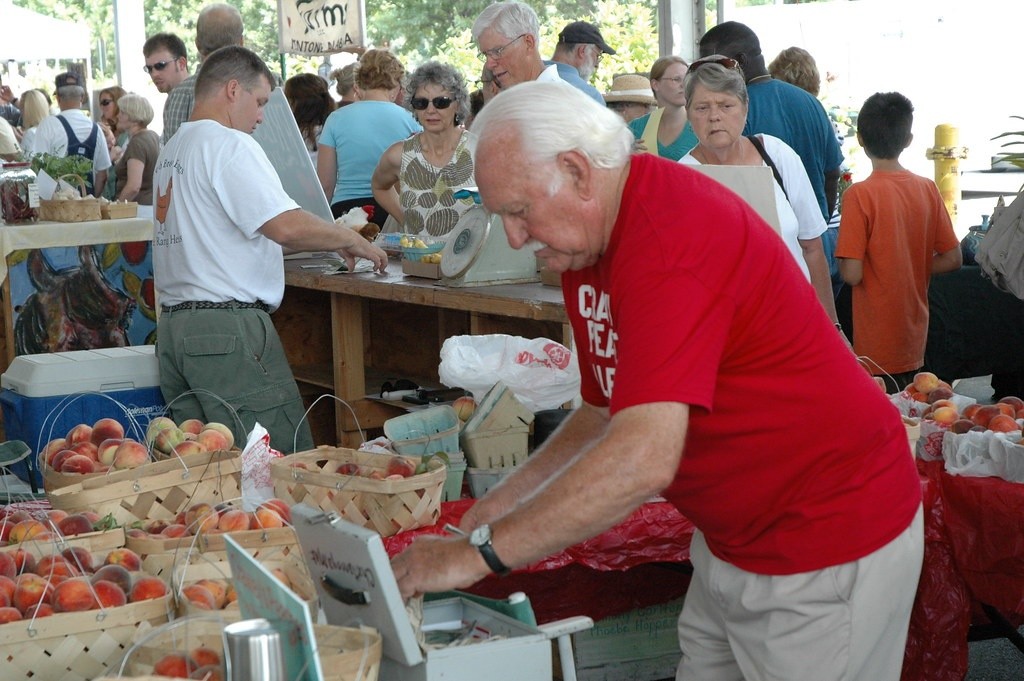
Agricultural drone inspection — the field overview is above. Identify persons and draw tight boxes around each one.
[389,77,924,681]
[32,72,112,199]
[676,54,854,356]
[315,47,424,239]
[834,92,962,397]
[371,61,484,239]
[152,47,388,456]
[0,0,844,282]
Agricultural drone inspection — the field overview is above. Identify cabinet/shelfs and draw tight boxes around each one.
[267,250,579,451]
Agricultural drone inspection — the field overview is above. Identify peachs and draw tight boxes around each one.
[284,454,416,479]
[0,498,291,681]
[40,418,149,474]
[871,371,1024,447]
[453,396,478,421]
[146,416,234,458]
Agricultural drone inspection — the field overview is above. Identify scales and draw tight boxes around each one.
[434,187,544,287]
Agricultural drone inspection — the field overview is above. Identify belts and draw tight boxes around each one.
[161,299,269,312]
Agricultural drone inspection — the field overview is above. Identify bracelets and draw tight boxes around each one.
[11,98,18,104]
[835,322,842,332]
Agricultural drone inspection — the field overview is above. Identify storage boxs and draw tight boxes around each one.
[0,345,175,493]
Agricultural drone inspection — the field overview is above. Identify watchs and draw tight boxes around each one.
[468,524,511,574]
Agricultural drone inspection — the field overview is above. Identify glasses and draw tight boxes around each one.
[660,76,682,84]
[689,58,738,71]
[591,47,604,62]
[143,57,178,74]
[476,34,525,64]
[100,99,114,106]
[410,97,456,110]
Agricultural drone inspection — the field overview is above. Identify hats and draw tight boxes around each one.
[558,20,616,54]
[53,72,83,95]
[604,75,654,104]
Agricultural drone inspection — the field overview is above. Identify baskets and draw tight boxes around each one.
[856,354,921,465]
[1,380,535,681]
[38,174,103,222]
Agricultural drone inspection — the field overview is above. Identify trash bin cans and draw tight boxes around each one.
[0,345,171,488]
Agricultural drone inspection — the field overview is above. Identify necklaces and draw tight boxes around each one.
[128,127,144,138]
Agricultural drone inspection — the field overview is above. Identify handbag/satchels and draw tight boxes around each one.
[440,332,579,413]
[974,181,1024,299]
